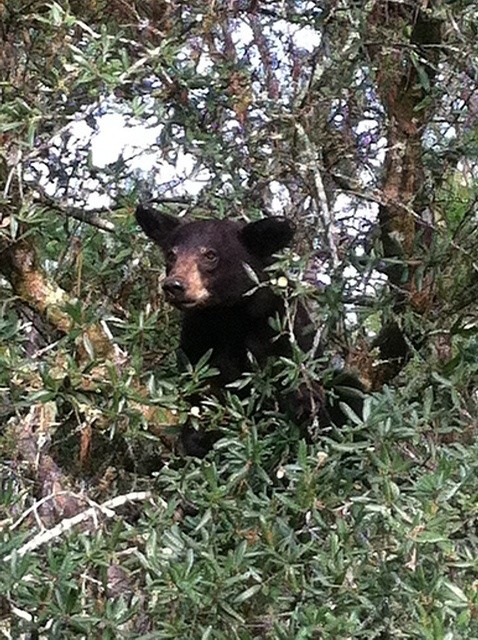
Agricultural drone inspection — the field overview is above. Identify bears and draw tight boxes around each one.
[134,203,372,468]
